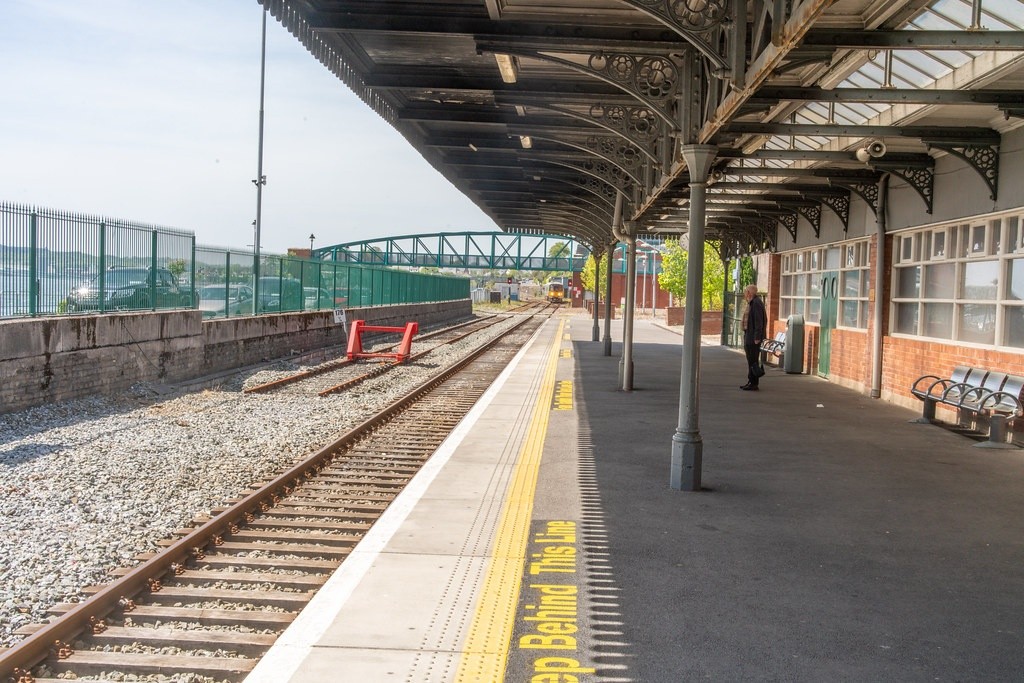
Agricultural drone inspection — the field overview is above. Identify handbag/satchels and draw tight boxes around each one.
[751,364,766,378]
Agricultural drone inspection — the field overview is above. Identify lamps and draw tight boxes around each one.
[495,54,519,83]
[519,136,532,149]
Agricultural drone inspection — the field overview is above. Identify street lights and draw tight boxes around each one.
[309,233,315,258]
[252,174,267,283]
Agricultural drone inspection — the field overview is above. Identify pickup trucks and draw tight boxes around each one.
[65,265,199,310]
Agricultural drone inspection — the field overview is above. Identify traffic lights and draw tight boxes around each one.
[568,279,572,286]
[507,277,512,283]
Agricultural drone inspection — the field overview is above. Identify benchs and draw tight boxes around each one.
[910,366,1024,450]
[761,332,788,368]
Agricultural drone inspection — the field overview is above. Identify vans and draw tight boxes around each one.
[248,277,304,311]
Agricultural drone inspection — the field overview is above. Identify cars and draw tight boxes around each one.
[303,288,334,309]
[329,286,375,304]
[200,284,262,316]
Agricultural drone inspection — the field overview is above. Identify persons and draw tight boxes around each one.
[739,285,767,390]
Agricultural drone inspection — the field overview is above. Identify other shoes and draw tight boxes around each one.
[743,382,759,391]
[740,379,752,389]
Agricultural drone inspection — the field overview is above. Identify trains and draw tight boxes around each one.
[520,282,564,303]
